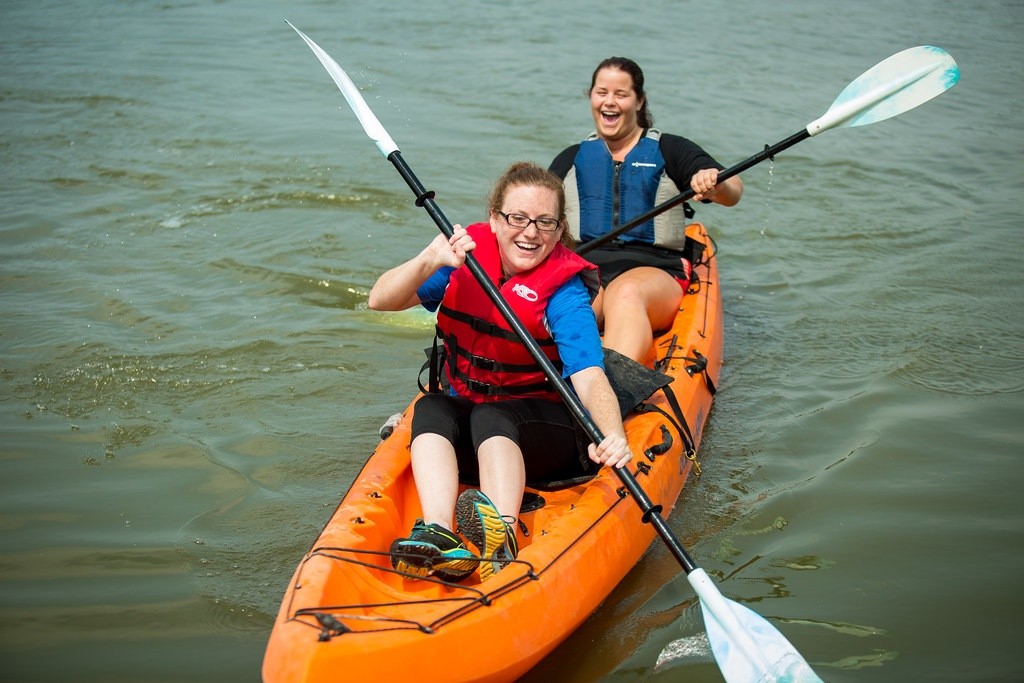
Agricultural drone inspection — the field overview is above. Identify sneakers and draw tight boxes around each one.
[390,518,480,583]
[455,489,519,585]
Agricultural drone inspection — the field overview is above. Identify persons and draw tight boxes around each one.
[546,55,744,372]
[368,160,634,581]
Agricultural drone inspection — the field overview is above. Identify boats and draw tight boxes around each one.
[260,222,723,682]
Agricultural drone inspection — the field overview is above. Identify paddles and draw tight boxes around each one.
[574,43,963,259]
[282,15,823,683]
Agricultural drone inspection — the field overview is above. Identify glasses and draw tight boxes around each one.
[499,210,565,232]
